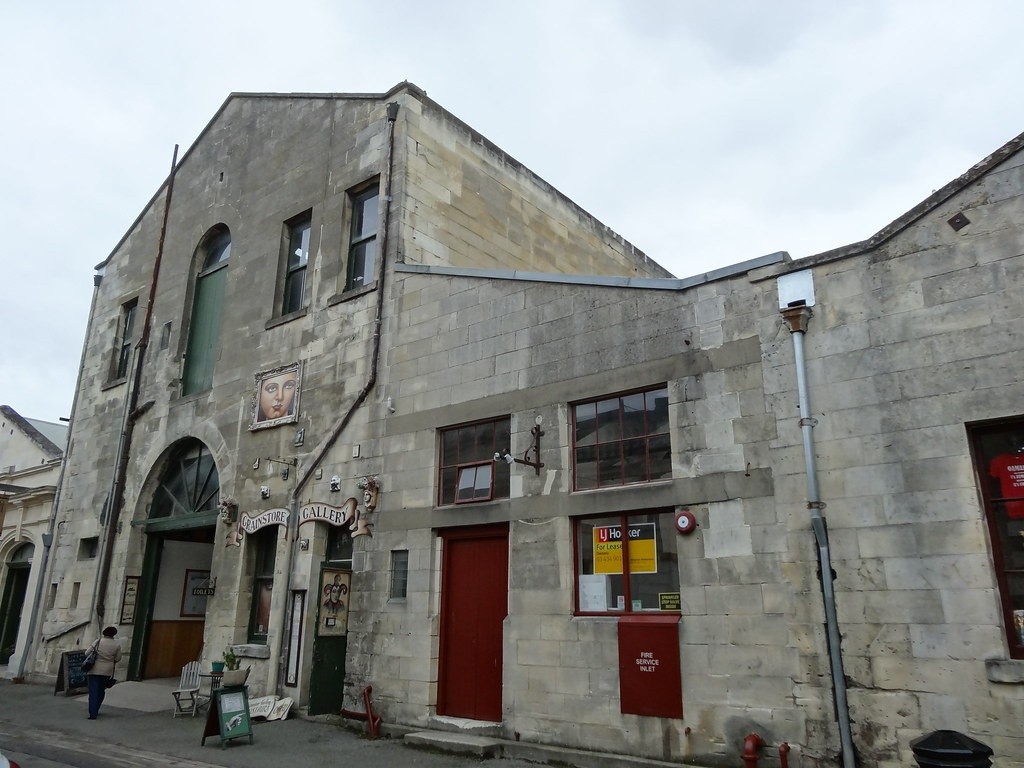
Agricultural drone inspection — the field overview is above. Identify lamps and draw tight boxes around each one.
[494,425,545,476]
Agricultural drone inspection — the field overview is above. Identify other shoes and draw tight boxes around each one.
[88,715,97,720]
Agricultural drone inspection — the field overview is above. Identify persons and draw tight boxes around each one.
[85,626,121,720]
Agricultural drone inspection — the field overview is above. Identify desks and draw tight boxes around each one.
[196,672,224,714]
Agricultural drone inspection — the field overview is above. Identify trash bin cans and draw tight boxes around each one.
[909,729,994,768]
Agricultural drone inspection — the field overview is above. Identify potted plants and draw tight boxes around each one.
[212,660,225,672]
[220,647,246,686]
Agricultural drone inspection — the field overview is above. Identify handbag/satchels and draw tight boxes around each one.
[81,651,98,672]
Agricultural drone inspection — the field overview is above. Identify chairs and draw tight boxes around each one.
[172,661,203,718]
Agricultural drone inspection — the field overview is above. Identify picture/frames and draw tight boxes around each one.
[284,589,308,688]
[318,571,350,636]
[247,362,304,431]
[179,569,211,617]
[120,576,141,624]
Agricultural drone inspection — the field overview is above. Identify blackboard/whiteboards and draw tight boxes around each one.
[62,648,90,695]
